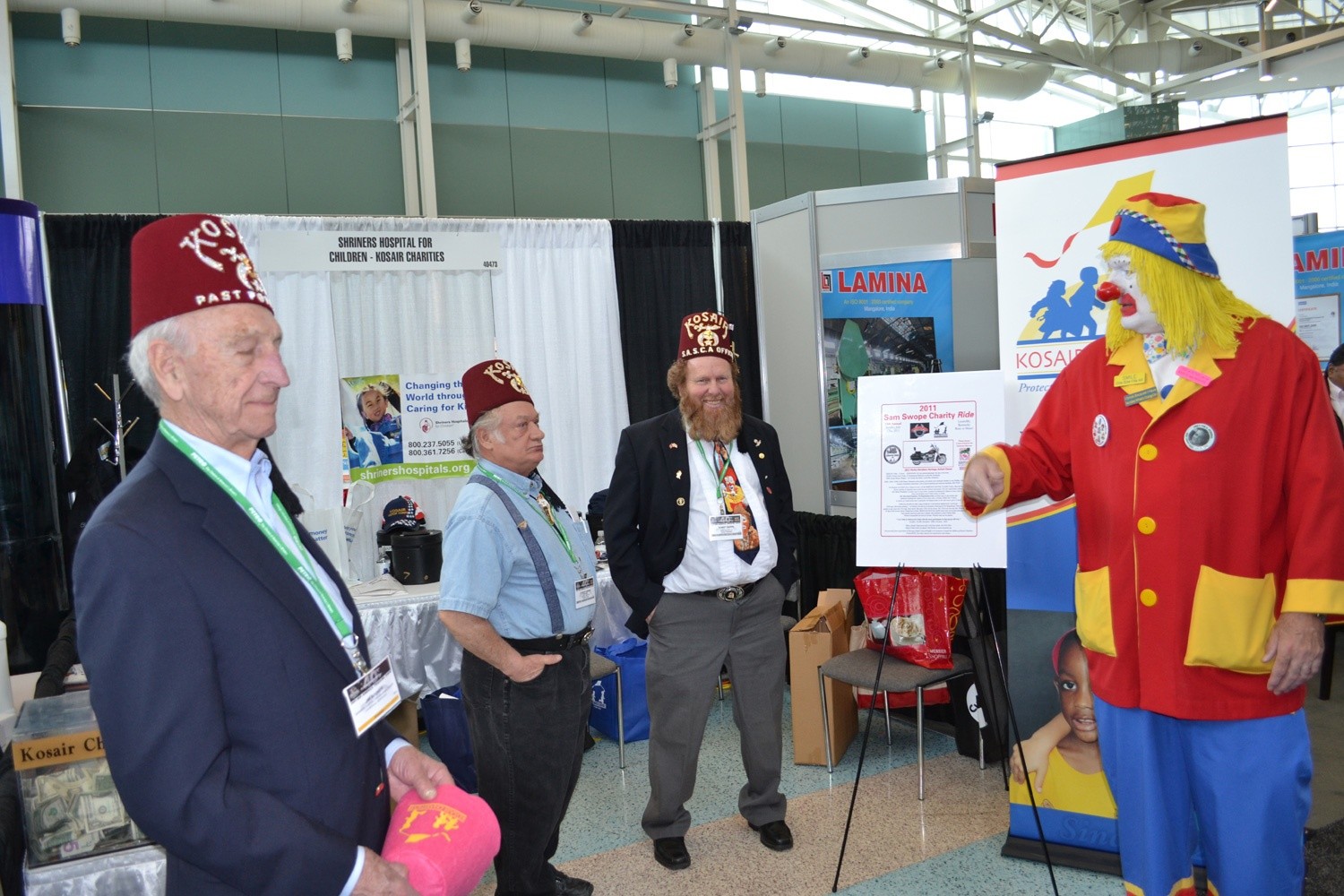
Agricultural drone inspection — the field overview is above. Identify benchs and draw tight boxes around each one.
[775,512,878,627]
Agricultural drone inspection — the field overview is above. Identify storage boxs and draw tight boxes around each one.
[10,689,163,866]
[787,585,861,769]
[19,844,169,896]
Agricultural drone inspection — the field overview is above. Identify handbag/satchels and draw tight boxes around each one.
[854,567,967,664]
[585,487,611,540]
[419,680,481,797]
[392,527,444,583]
[589,639,653,745]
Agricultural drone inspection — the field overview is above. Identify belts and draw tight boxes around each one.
[695,577,765,603]
[502,621,596,658]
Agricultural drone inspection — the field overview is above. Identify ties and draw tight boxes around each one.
[709,437,762,566]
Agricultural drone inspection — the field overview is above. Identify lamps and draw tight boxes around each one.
[921,58,944,75]
[662,59,678,89]
[344,0,358,12]
[462,0,483,24]
[573,11,592,32]
[335,29,354,63]
[60,9,81,48]
[764,36,787,55]
[849,46,870,65]
[674,23,694,45]
[455,39,471,71]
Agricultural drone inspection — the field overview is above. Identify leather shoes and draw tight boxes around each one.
[653,837,691,870]
[547,867,594,895]
[748,817,793,852]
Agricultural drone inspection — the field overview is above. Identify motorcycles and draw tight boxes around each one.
[909,444,947,466]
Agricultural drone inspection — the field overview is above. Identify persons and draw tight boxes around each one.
[604,337,802,870]
[73,274,456,895]
[1322,343,1344,444]
[1008,622,1118,819]
[963,195,1344,896]
[341,382,403,469]
[436,391,598,896]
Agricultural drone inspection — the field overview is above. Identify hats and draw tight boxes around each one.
[462,361,535,426]
[383,784,503,896]
[1112,193,1221,279]
[123,213,276,337]
[676,309,735,364]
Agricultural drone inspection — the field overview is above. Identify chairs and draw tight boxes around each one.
[586,650,626,772]
[817,649,986,802]
